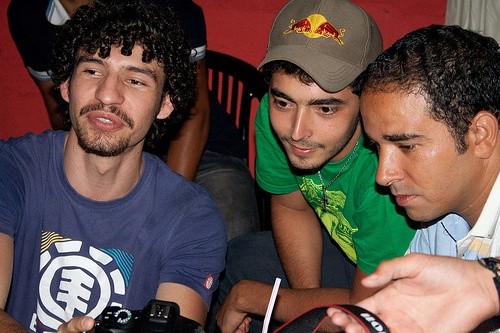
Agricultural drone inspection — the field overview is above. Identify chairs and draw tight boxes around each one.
[201,49,271,176]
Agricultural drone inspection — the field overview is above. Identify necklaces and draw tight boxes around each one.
[317,132,368,215]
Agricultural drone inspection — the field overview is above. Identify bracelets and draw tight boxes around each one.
[480,257,500,304]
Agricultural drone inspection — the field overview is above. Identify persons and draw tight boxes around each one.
[351,24,500,333]
[214,1,421,333]
[6,0,210,181]
[327,253,500,332]
[1,1,226,333]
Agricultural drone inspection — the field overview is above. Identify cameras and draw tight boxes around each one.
[94,299,202,333]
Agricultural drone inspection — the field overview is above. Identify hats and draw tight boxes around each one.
[257,0,383,94]
